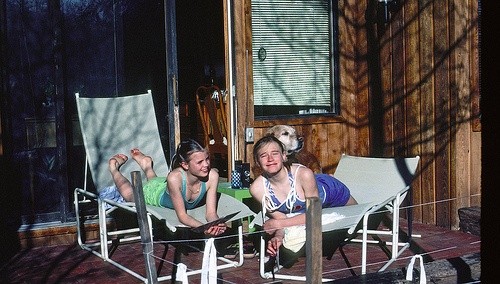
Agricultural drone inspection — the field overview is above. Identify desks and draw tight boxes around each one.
[199,99,230,148]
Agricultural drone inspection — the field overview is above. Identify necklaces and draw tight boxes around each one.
[187,182,202,195]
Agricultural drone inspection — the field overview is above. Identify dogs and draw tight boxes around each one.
[251,125,320,179]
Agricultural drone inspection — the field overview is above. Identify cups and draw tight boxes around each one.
[230,160,251,189]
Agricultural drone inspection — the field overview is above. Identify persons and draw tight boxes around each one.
[108,137,227,236]
[249,135,358,257]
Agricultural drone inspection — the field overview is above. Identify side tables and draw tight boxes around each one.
[216,180,261,250]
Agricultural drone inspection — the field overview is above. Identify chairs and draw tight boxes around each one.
[248,153,421,284]
[72,87,255,283]
[195,84,229,171]
